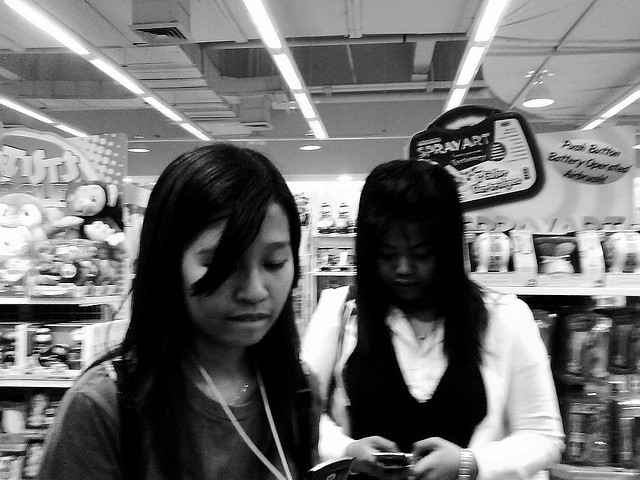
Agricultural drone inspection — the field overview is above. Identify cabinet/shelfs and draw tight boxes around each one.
[1,293,131,390]
[305,233,358,308]
[470,271,640,479]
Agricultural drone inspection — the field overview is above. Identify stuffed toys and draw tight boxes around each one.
[474,223,519,278]
[66,180,126,247]
[27,327,75,363]
[0,193,85,285]
[22,397,54,431]
[332,201,350,233]
[316,202,335,234]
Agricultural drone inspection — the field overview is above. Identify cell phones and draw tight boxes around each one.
[306,456,357,480]
[370,452,413,466]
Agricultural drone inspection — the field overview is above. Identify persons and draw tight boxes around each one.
[299,158,567,480]
[34,142,320,480]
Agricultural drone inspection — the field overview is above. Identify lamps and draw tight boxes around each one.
[0,96,89,138]
[242,1,329,141]
[523,74,554,109]
[127,137,151,154]
[1,1,216,142]
[299,133,321,151]
[577,82,640,129]
[442,0,512,111]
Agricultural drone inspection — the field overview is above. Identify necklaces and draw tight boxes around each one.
[413,322,435,342]
[226,375,253,405]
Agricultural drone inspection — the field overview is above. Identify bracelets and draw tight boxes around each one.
[458,448,474,480]
